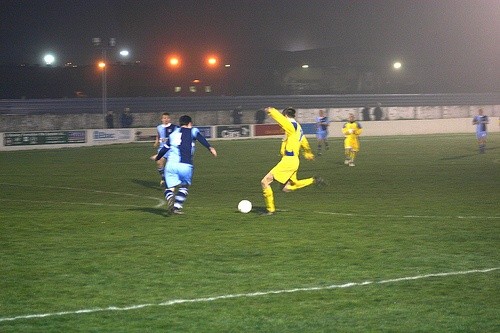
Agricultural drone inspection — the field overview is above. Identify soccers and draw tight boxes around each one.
[237,199,253,214]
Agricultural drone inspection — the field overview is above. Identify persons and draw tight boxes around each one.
[153,113,180,192]
[362,103,372,120]
[155,115,217,215]
[255,107,266,123]
[119,107,133,128]
[105,109,115,128]
[260,106,330,216]
[473,109,489,153]
[373,102,383,120]
[316,110,329,157]
[342,113,363,167]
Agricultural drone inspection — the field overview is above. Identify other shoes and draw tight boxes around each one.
[261,210,276,216]
[312,176,322,185]
[344,158,352,164]
[349,162,356,167]
[160,180,166,187]
[172,207,186,215]
[167,200,176,215]
